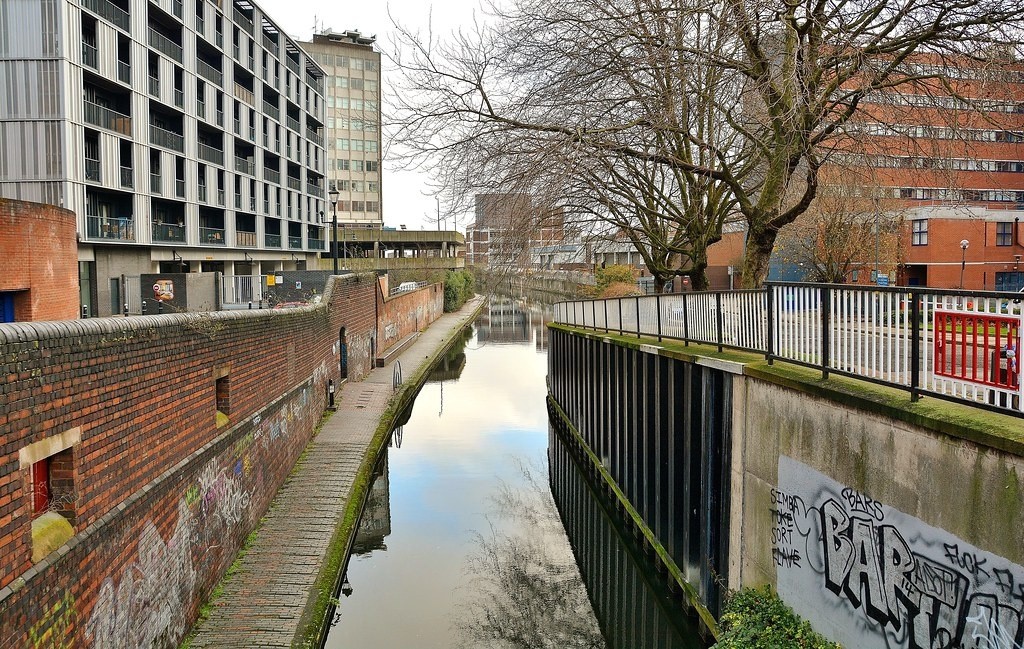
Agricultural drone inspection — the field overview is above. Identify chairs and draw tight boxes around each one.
[99,225,118,239]
[169,230,174,241]
[208,233,223,244]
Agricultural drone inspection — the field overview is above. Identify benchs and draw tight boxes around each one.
[377,331,419,367]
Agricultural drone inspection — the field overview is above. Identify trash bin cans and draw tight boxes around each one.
[989,345,1019,409]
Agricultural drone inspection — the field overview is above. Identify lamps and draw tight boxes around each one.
[329,385,335,394]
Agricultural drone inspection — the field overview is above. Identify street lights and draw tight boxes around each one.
[328,184,340,275]
[957,239,970,307]
[1013,254,1023,292]
